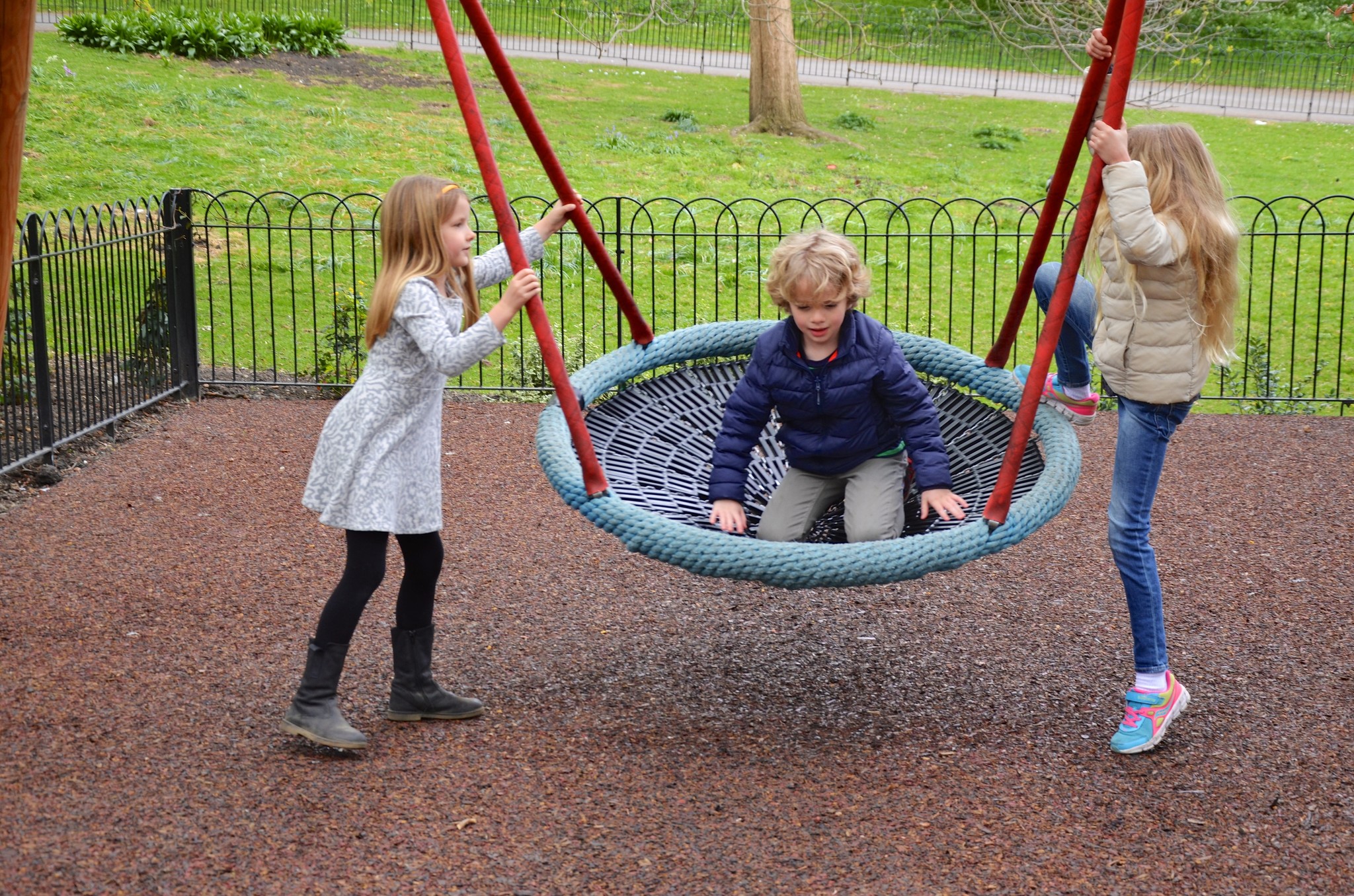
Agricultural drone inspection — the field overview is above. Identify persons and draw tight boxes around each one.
[1014,27,1243,754]
[282,176,583,749]
[707,230,969,544]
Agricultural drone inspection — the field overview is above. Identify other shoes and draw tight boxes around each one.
[905,459,913,501]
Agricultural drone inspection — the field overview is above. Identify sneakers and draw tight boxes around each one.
[1013,365,1098,425]
[1110,670,1191,754]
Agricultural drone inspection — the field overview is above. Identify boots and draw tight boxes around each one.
[387,622,486,721]
[278,636,369,748]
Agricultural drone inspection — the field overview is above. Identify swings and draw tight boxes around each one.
[421,1,1149,592]
